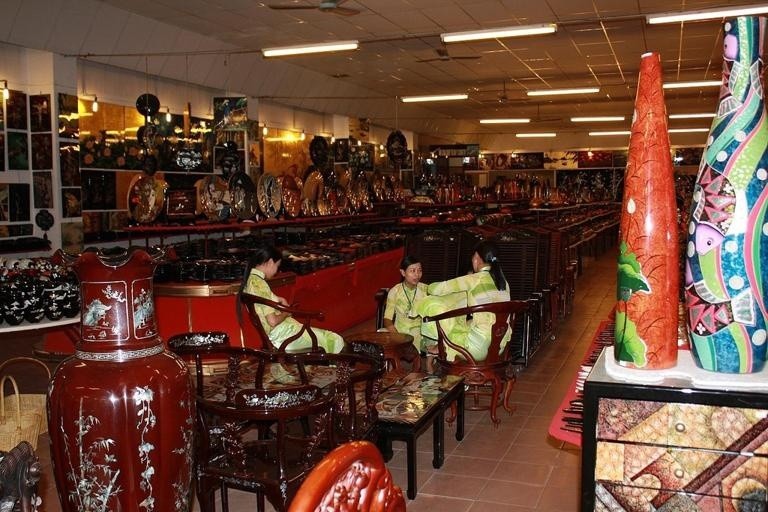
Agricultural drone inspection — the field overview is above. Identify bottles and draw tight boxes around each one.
[529,186,563,208]
[496,185,501,200]
[521,184,525,198]
[353,145,373,169]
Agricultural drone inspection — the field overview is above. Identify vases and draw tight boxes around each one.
[44,244,199,511]
[684,15,767,372]
[615,52,680,370]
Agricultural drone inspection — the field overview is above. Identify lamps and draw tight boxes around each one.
[261,4,766,59]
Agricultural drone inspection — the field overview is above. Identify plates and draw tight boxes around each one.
[129,175,164,224]
[200,170,404,222]
[309,136,329,167]
[386,130,408,165]
[176,148,203,170]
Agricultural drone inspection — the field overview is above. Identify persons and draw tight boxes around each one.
[236,245,345,354]
[375,256,429,356]
[408,240,513,362]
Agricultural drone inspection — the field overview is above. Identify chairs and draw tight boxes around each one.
[181,331,466,512]
[424,300,527,427]
[239,292,330,365]
